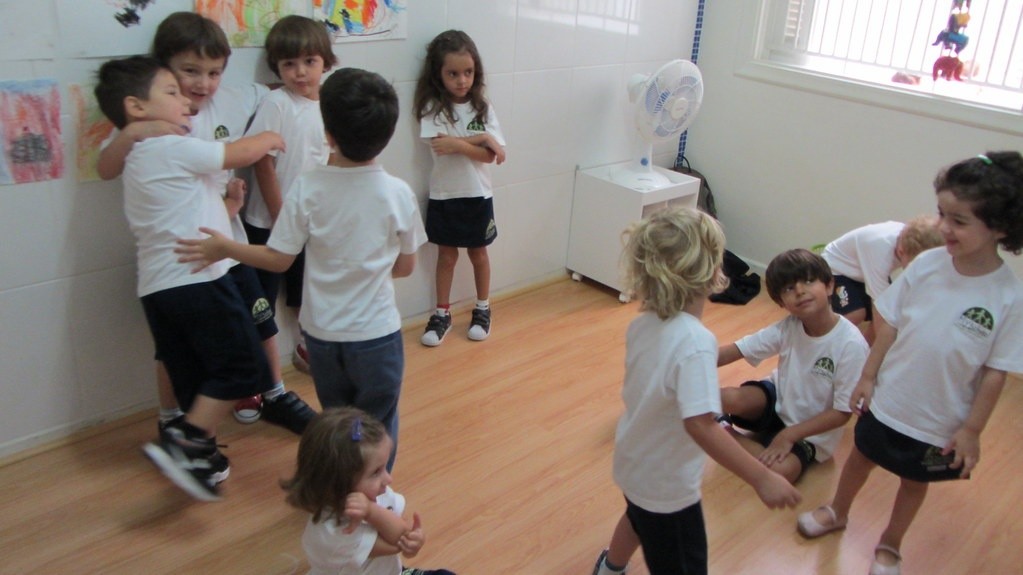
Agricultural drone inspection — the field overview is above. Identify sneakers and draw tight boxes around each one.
[261,388,322,434]
[420,311,452,347]
[466,305,492,341]
[142,422,220,504]
[158,413,232,481]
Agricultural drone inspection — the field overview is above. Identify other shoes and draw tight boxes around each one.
[291,344,310,375]
[797,504,847,538]
[234,392,263,423]
[869,544,903,575]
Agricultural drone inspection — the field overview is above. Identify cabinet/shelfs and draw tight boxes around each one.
[565,159,702,301]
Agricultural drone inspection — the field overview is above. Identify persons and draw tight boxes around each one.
[796,150,1022,575]
[412,29,506,347]
[171,66,428,510]
[92,10,428,501]
[593,206,802,575]
[819,214,946,326]
[717,249,871,486]
[276,406,456,575]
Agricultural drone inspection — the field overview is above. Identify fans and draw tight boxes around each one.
[625,58,704,189]
